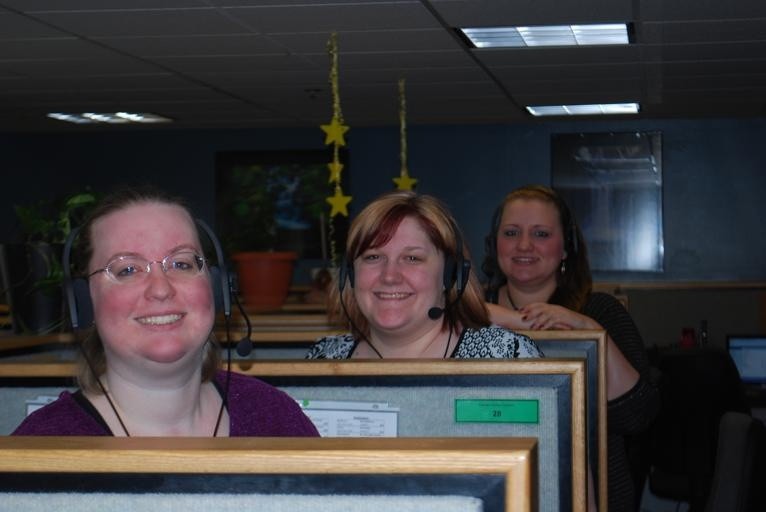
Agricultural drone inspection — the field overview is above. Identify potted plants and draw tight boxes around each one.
[5,196,93,336]
[214,164,313,313]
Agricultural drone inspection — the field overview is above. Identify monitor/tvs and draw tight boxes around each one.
[726,333,766,384]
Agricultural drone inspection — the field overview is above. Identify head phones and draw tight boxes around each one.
[484,201,582,262]
[62,213,245,330]
[338,213,471,294]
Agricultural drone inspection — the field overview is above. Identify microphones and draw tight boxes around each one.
[428,286,465,320]
[232,295,253,357]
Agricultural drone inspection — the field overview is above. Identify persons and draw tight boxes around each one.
[7,181,323,437]
[304,190,545,360]
[482,183,663,511]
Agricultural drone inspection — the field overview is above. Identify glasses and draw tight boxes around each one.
[85,252,209,284]
